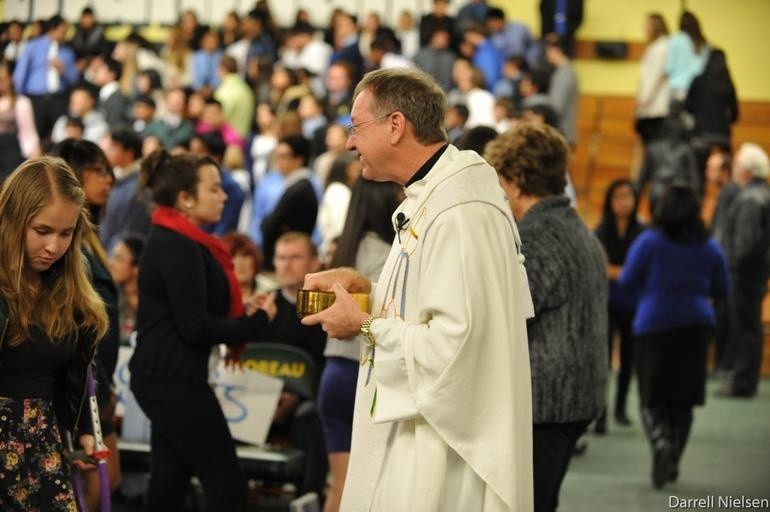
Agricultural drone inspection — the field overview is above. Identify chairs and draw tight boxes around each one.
[102,344,152,461]
[207,337,316,500]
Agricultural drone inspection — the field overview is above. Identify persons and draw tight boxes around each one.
[130,148,278,510]
[482,121,610,512]
[318,175,405,510]
[0,156,110,510]
[1,3,769,488]
[299,66,539,510]
